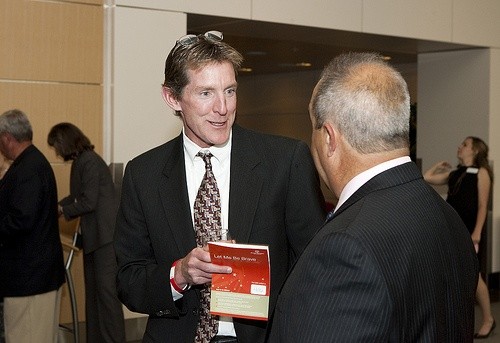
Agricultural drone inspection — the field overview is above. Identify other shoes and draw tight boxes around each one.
[477,323,496,338]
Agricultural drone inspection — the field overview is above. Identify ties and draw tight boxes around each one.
[194,152,223,343]
[324,209,334,221]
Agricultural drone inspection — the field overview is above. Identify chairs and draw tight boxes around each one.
[59,214,80,343]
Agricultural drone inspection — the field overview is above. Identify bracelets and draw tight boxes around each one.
[171,258,191,293]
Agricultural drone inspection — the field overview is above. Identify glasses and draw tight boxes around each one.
[171,30,224,57]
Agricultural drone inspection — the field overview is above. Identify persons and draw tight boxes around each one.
[114,34,328,343]
[46,122,128,343]
[423,136,497,338]
[265,48,475,343]
[0,111,65,343]
